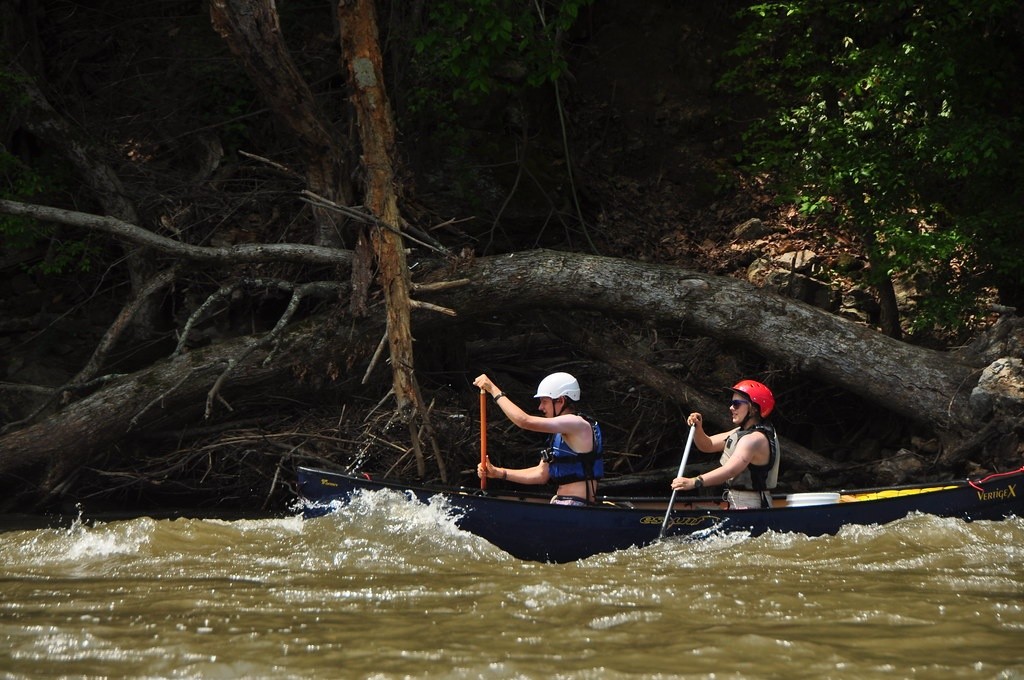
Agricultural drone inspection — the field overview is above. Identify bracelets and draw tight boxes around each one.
[493,392,507,404]
[501,467,507,480]
[697,476,705,483]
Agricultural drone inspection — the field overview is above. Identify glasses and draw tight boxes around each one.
[730,399,749,408]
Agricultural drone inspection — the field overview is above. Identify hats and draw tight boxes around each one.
[732,380,774,417]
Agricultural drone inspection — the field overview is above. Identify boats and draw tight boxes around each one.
[296,464,1024,565]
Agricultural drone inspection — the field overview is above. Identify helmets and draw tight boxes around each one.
[534,372,580,402]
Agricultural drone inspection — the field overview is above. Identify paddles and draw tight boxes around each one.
[655,424,696,541]
[480,390,488,490]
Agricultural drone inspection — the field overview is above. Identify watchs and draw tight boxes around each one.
[693,477,704,490]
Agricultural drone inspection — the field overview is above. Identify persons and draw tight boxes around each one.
[671,380,780,509]
[473,372,603,507]
